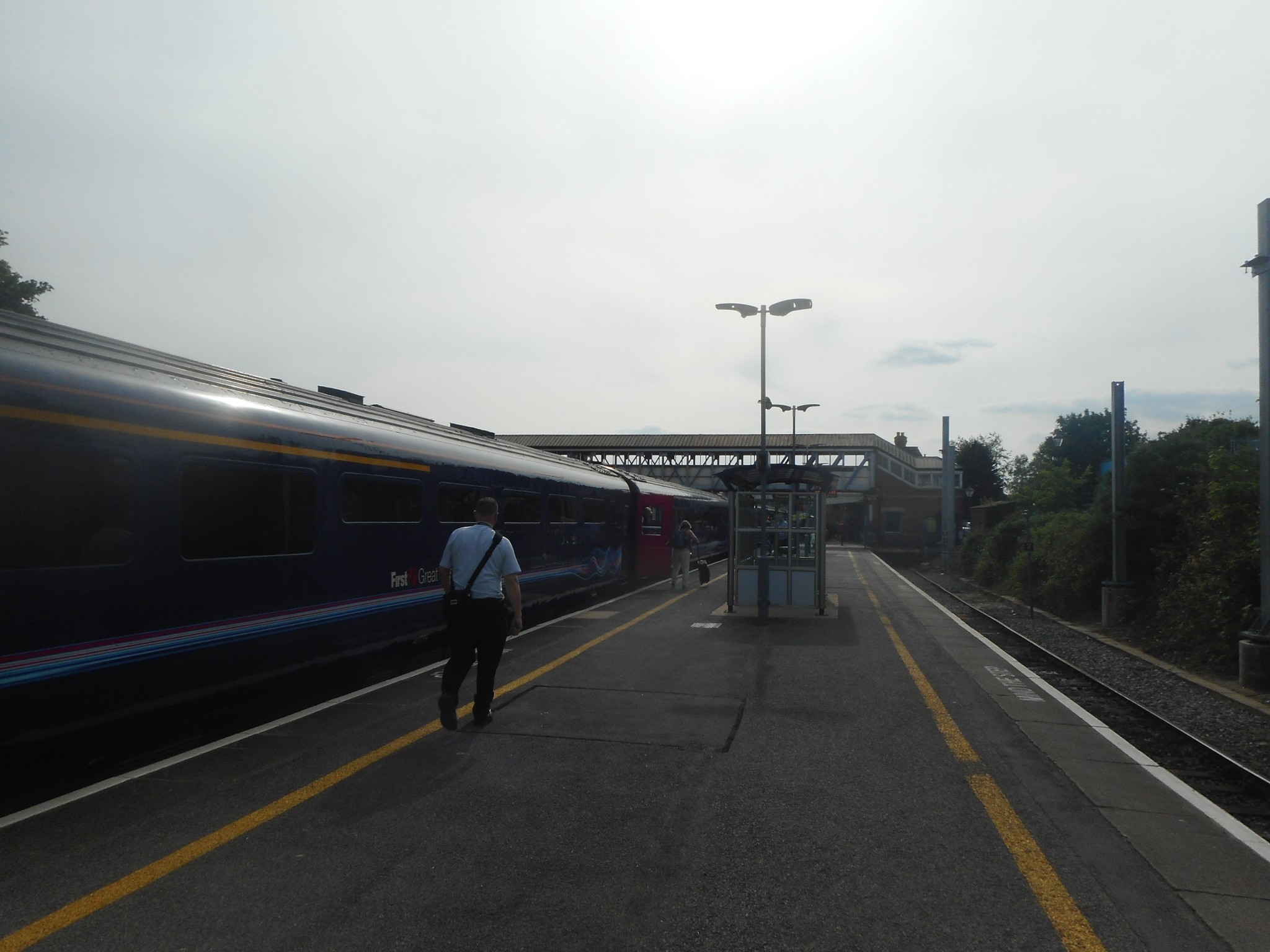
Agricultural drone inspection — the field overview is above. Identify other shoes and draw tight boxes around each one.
[683,584,689,588]
[671,582,675,587]
[438,693,459,732]
[473,711,494,726]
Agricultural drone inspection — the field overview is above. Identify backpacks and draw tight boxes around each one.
[672,528,689,549]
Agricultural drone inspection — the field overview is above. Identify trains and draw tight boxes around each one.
[1,305,735,775]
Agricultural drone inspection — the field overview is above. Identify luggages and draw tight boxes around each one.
[696,543,710,585]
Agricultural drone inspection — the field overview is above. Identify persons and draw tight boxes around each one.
[670,520,700,590]
[436,493,524,729]
[771,511,790,548]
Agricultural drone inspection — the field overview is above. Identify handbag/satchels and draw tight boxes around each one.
[444,590,473,630]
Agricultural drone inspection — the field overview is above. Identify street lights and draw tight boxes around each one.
[715,297,813,628]
[768,403,820,559]
